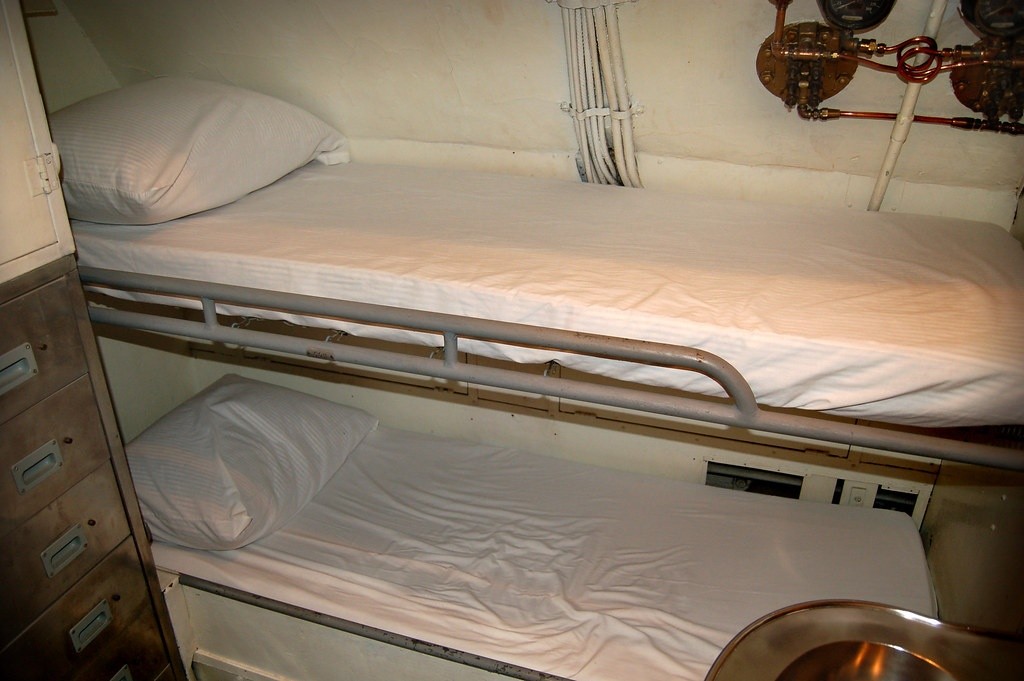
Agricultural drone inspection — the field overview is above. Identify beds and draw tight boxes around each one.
[70,143,1024,681]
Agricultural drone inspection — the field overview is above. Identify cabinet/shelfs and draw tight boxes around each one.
[0,251,188,681]
[0,0,77,283]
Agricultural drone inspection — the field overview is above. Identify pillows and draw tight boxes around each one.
[124,373,381,550]
[46,78,350,226]
